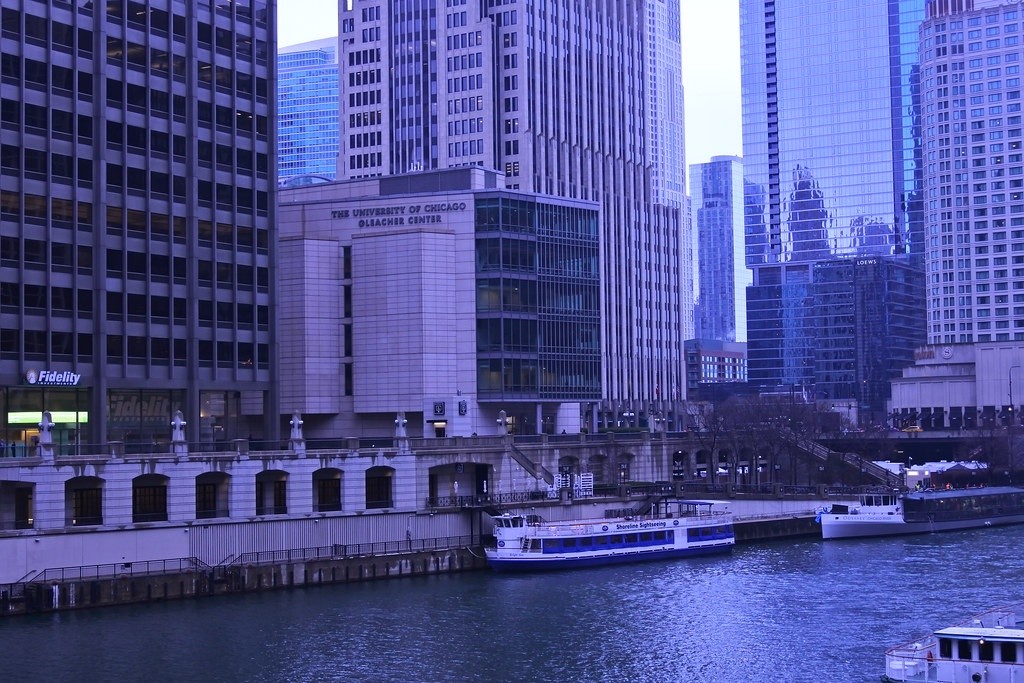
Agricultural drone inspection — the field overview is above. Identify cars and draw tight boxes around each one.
[902,426,923,432]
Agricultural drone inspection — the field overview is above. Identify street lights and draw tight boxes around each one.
[1008,365,1021,404]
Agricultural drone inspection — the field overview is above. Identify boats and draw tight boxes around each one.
[482,502,737,571]
[818,486,1024,540]
[879,604,1024,682]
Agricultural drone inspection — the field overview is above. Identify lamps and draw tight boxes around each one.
[430,514,434,519]
[315,519,319,524]
[531,507,535,511]
[184,528,188,533]
[725,505,728,510]
[593,503,597,506]
[35,536,40,543]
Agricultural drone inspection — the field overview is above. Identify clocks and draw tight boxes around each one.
[26,368,38,384]
